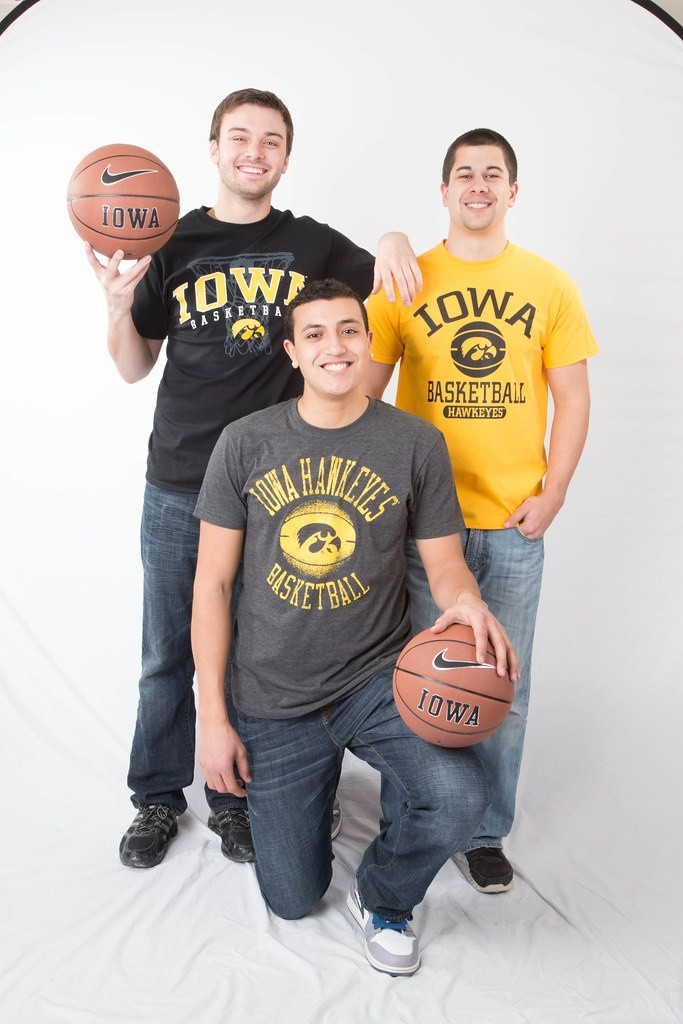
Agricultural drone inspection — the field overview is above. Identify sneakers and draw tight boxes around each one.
[119,803,178,868]
[450,847,514,894]
[329,795,343,840]
[347,866,421,977]
[207,808,256,863]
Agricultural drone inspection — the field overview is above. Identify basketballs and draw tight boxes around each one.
[392,624,515,749]
[67,144,181,261]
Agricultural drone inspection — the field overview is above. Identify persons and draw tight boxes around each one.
[363,128,590,895]
[84,88,424,869]
[190,278,522,974]
